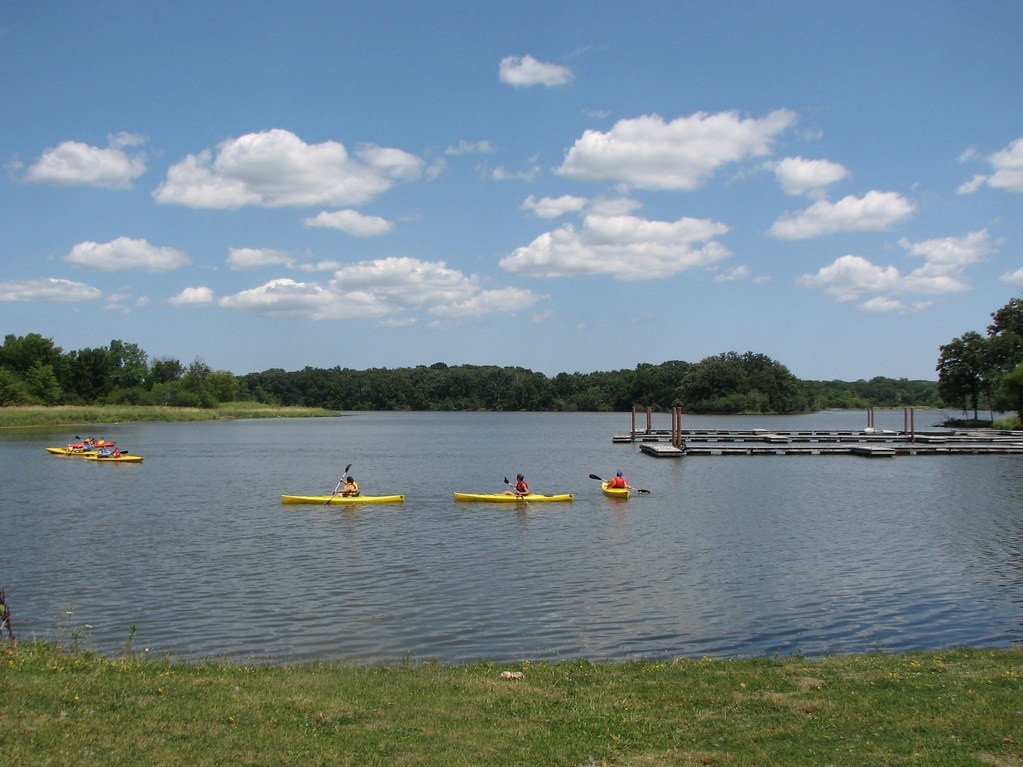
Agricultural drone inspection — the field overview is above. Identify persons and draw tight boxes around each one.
[112,448,122,457]
[607,471,631,490]
[86,436,97,445]
[82,440,93,451]
[97,437,105,445]
[503,473,529,497]
[334,476,361,498]
[98,446,111,456]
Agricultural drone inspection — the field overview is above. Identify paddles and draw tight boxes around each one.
[323,464,353,503]
[590,474,652,494]
[503,477,525,500]
[75,436,84,441]
[120,450,129,454]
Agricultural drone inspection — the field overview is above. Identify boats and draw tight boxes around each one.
[453,492,574,503]
[281,494,405,504]
[68,441,116,448]
[601,481,630,499]
[46,447,98,455]
[83,455,144,463]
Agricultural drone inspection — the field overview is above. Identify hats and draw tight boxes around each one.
[617,471,623,477]
